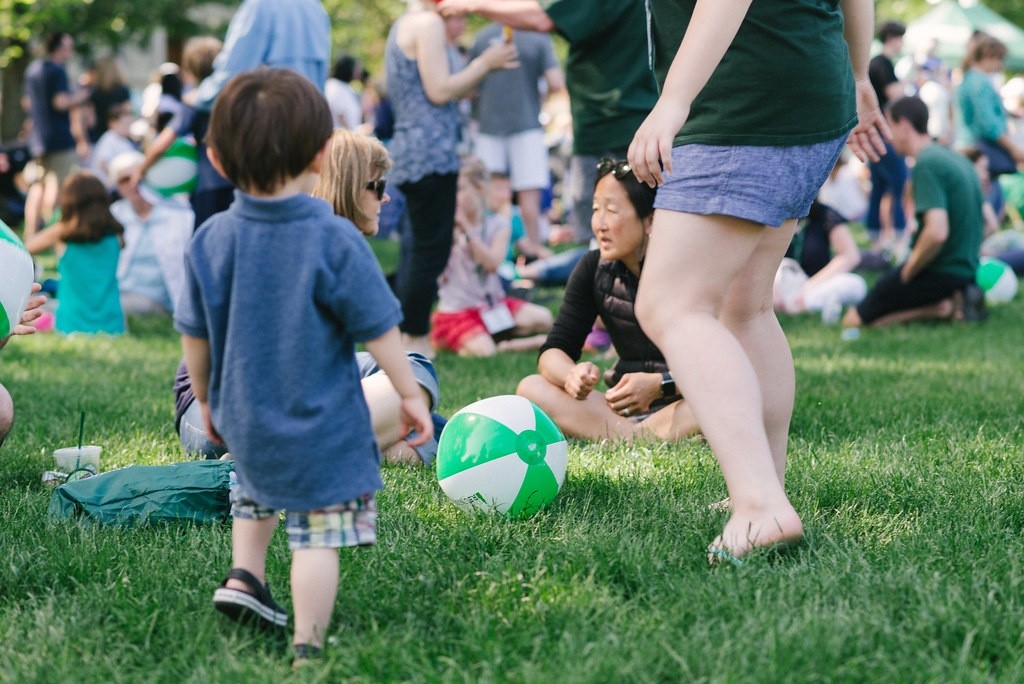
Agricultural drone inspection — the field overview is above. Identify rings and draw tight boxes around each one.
[624,408,630,417]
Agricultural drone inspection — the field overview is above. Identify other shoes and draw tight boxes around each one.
[953,284,986,326]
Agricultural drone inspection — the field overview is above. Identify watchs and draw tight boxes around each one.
[660,370,677,401]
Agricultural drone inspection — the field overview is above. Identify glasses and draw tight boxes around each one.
[364,180,386,200]
[595,157,631,180]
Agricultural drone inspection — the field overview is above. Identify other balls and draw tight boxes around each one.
[145,135,200,204]
[972,259,1020,310]
[435,394,573,524]
[0,216,35,341]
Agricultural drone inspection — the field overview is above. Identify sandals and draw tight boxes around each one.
[290,644,322,670]
[213,571,288,631]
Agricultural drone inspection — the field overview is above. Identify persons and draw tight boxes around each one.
[431,156,557,355]
[626,0,894,564]
[0,0,1023,325]
[174,69,434,670]
[14,159,132,338]
[517,159,702,446]
[174,126,449,465]
[0,283,46,445]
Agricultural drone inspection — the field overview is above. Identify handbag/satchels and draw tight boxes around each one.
[47,458,231,527]
